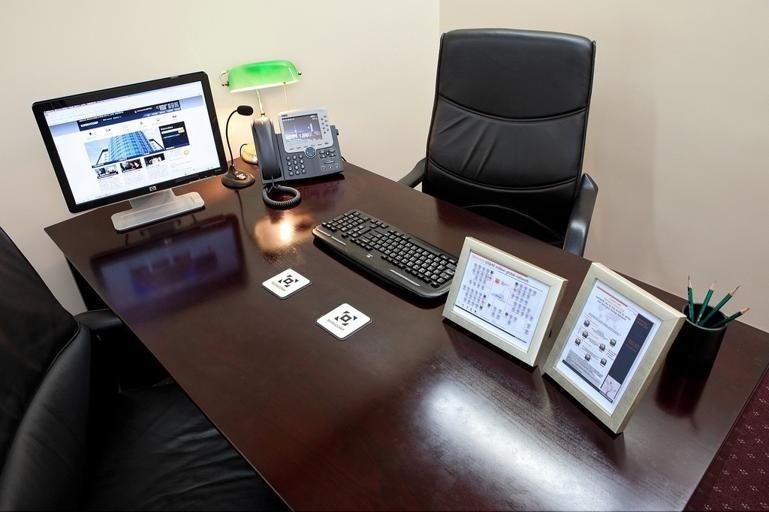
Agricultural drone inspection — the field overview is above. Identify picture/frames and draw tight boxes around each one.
[543,262,688,434]
[442,237,569,367]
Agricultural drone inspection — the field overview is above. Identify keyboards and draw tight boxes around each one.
[313,208,456,305]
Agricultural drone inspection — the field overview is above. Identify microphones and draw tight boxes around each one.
[223,104,255,188]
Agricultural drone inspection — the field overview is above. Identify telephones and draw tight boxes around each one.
[251,107,344,209]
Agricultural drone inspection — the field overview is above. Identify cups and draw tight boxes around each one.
[656,302,728,417]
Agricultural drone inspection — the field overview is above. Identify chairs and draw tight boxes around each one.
[0,226,293,511]
[397,28,599,258]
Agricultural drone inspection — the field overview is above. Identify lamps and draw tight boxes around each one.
[220,60,301,163]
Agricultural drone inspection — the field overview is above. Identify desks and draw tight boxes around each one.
[44,156,768,511]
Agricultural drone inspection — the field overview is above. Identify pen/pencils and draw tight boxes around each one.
[695,280,716,325]
[712,307,750,327]
[700,285,740,325]
[687,276,695,323]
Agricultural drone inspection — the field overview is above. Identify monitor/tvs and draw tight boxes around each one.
[29,70,227,233]
